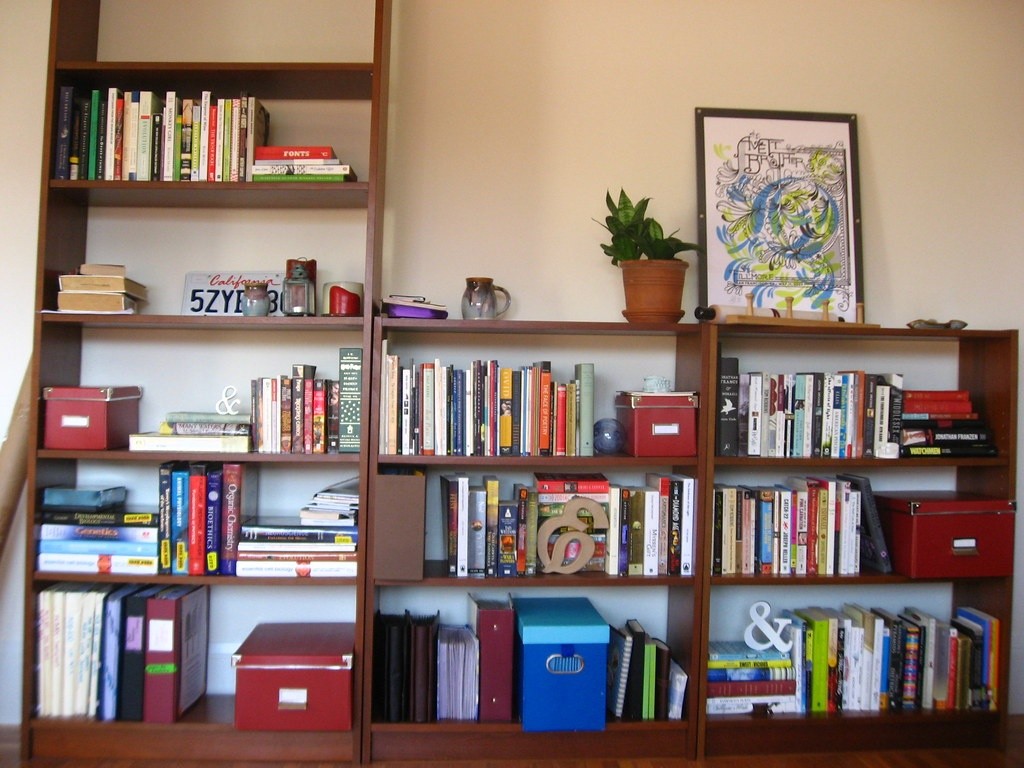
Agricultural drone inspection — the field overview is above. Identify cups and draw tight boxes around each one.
[461,277,512,320]
[321,282,363,317]
[329,285,360,317]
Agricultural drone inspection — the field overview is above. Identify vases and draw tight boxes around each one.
[459,275,510,321]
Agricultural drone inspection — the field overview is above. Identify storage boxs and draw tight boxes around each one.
[43,385,140,450]
[614,393,696,457]
[868,489,1016,578]
[509,597,611,732]
[229,622,354,733]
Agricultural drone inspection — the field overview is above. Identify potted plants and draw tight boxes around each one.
[598,188,693,325]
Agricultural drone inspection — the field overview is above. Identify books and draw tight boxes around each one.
[54,84,359,181]
[125,346,361,455]
[711,475,895,576]
[35,460,360,578]
[377,337,703,579]
[34,583,208,724]
[55,261,150,313]
[714,355,999,457]
[706,601,1001,710]
[372,591,689,724]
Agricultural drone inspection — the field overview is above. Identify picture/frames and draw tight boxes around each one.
[692,104,866,324]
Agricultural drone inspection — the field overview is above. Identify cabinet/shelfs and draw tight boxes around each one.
[1,1,1017,767]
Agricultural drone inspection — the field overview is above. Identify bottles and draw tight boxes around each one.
[240,282,271,317]
